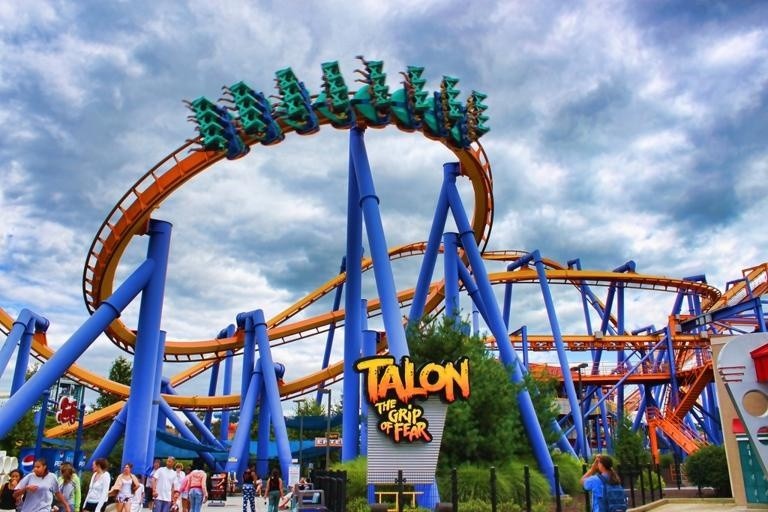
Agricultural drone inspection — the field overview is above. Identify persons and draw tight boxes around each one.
[0,452,209,512]
[581,453,621,512]
[241,464,309,511]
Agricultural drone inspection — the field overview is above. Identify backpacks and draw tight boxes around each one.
[597,474,628,512]
